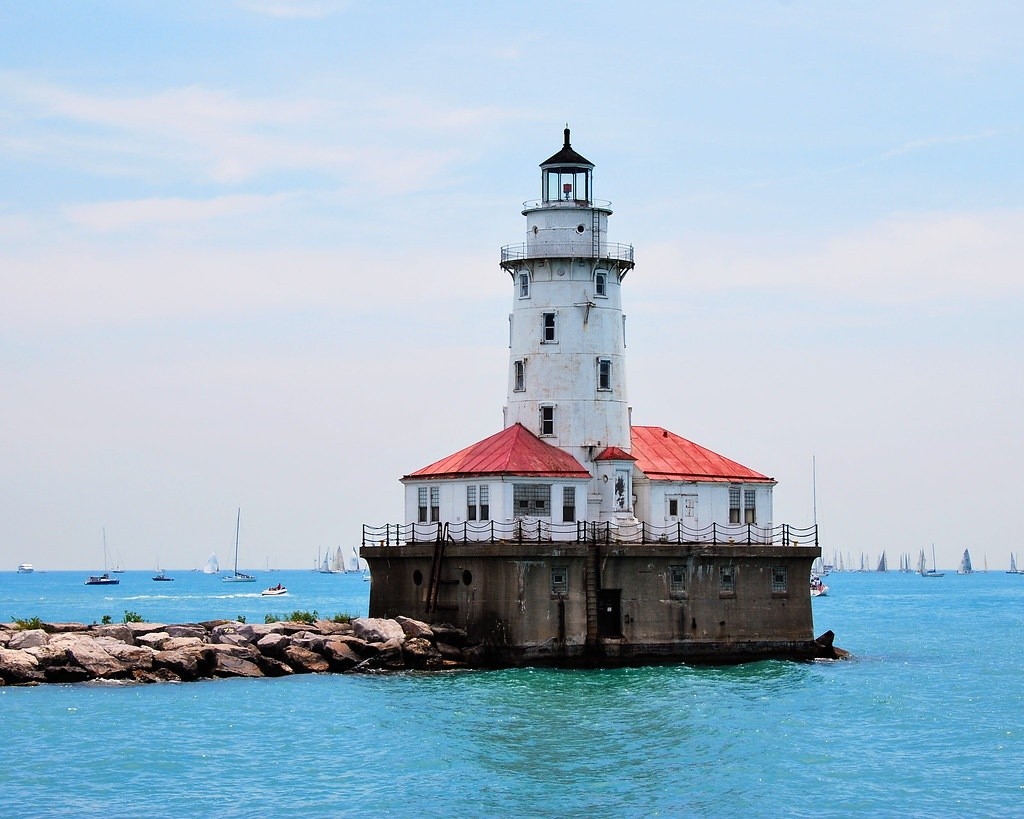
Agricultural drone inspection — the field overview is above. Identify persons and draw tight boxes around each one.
[276,583,282,591]
[810,577,822,590]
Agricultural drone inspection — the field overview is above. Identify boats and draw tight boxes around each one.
[810,573,829,596]
[16,563,34,574]
[110,566,127,574]
[260,587,288,596]
[151,573,175,582]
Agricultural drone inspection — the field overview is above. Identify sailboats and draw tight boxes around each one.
[311,543,372,582]
[203,551,221,574]
[811,546,928,575]
[83,527,120,585]
[221,505,258,582]
[262,555,275,571]
[921,544,946,577]
[1005,552,1023,574]
[809,455,829,577]
[956,547,975,576]
